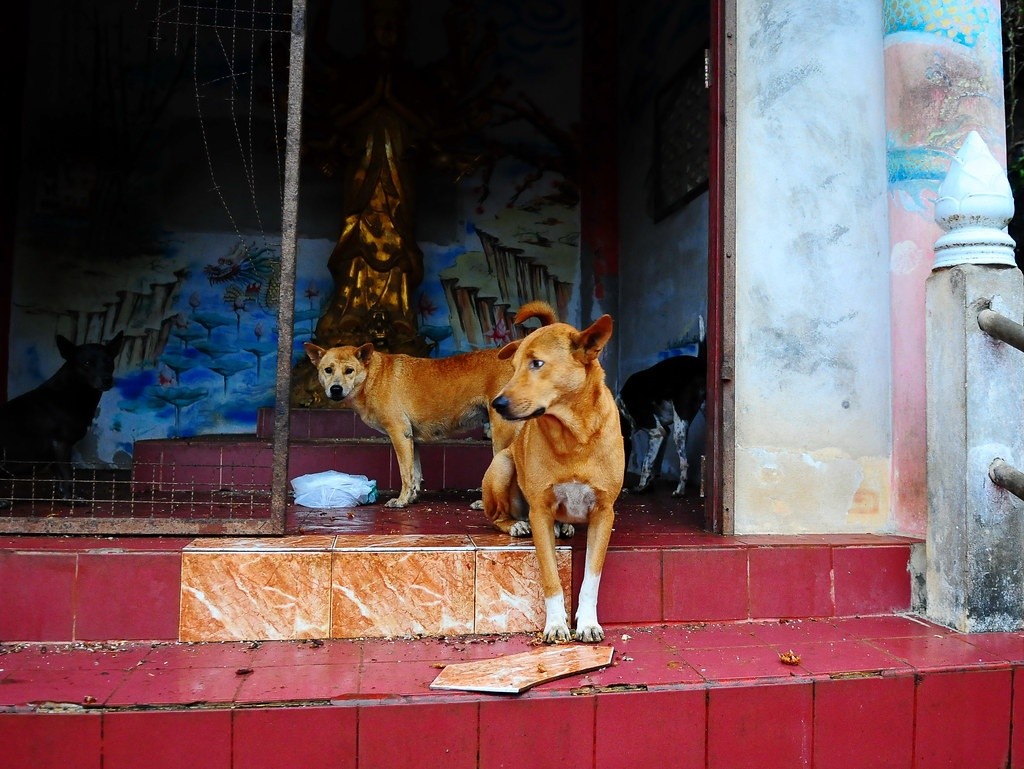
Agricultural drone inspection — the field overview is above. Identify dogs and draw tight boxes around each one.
[0,329,127,511]
[302,343,528,511]
[482,299,626,644]
[614,314,707,497]
